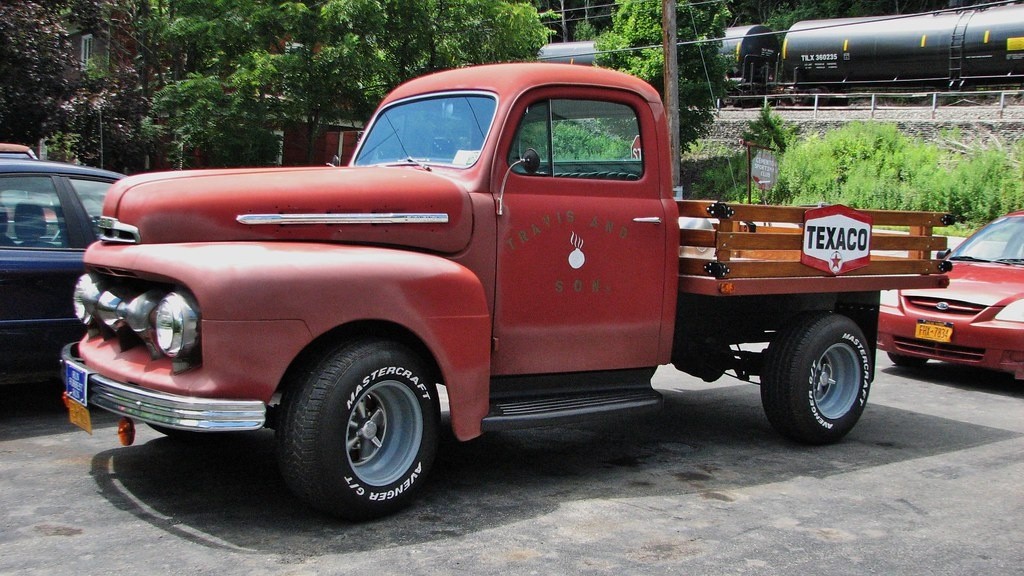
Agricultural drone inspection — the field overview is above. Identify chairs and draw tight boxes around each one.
[0,204,16,246]
[14,203,55,247]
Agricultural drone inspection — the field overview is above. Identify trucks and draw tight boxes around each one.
[57,61,954,521]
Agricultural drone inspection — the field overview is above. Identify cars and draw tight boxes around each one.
[875,209,1024,380]
[0,158,130,363]
[0,143,59,239]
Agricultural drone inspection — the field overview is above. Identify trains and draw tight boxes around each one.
[537,0,1024,107]
